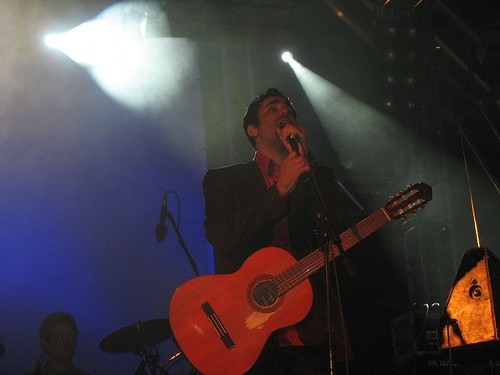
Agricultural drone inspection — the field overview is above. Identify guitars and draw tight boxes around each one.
[169,182,433,375]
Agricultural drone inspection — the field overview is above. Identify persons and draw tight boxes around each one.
[28,312,85,375]
[203,88,377,375]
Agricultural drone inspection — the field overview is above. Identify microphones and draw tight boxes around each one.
[277,122,299,151]
[155,193,168,242]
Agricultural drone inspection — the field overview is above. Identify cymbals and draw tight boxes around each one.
[98,317,172,354]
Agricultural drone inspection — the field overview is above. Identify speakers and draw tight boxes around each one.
[439,246,500,350]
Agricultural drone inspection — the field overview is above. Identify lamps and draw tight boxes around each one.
[378,0,442,124]
